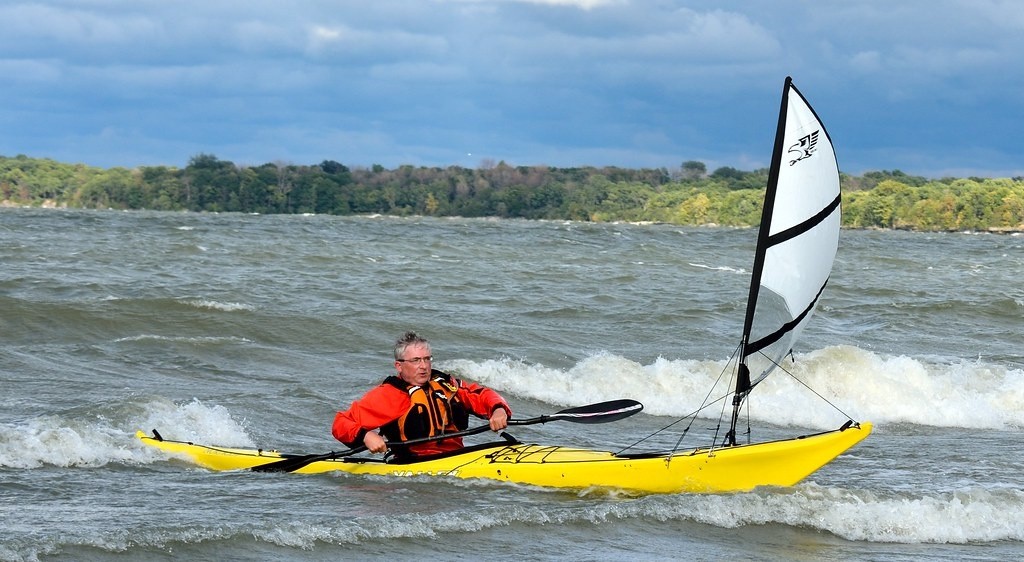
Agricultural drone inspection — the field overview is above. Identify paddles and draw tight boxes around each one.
[217,398,645,476]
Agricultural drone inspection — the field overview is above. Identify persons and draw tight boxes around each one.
[331,331,512,456]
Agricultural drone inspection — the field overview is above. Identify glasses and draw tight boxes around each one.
[398,355,434,364]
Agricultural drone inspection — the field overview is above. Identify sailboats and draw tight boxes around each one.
[133,78,874,498]
